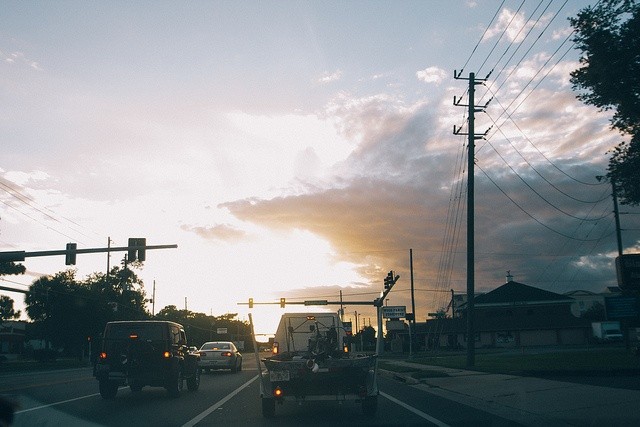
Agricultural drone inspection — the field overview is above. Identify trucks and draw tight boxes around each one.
[248,307,383,415]
[592,320,623,343]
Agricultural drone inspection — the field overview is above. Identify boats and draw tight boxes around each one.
[260,327,378,390]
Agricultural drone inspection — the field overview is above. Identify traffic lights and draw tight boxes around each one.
[249,298,253,308]
[387,271,394,284]
[384,276,389,289]
[281,298,285,307]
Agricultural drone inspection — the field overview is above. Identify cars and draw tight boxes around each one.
[198,342,242,374]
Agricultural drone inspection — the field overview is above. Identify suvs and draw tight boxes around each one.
[93,320,201,400]
